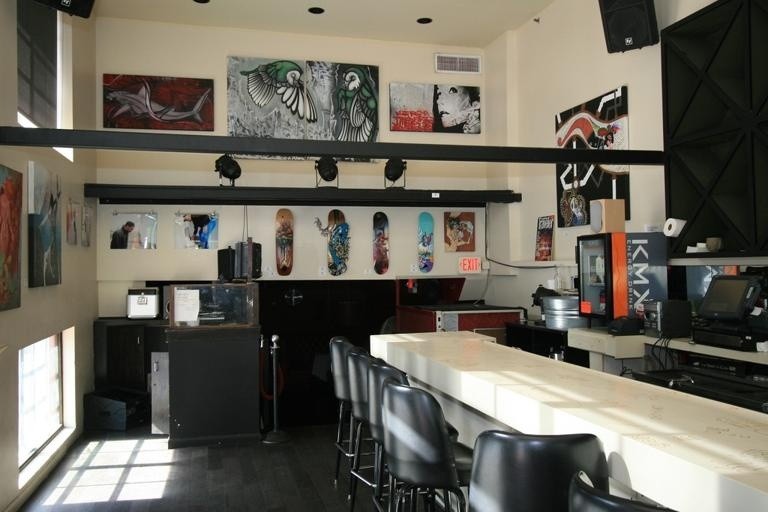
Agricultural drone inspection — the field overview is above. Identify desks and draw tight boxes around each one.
[368,329,768,512]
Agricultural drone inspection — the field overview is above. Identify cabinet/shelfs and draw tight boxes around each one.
[149,327,261,449]
[93,321,146,395]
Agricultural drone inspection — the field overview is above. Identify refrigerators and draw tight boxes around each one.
[575,230,669,325]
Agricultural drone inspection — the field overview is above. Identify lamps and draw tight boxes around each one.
[213,152,242,188]
[381,156,411,191]
[312,153,341,191]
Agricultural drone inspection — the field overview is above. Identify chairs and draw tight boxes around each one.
[501,321,565,358]
[568,469,676,512]
[326,334,412,510]
[378,377,472,510]
[466,429,609,512]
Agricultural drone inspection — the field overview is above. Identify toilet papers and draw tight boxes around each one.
[663,217,689,238]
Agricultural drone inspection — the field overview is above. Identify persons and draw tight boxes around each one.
[433,85,480,134]
[110,221,134,248]
[184,214,216,248]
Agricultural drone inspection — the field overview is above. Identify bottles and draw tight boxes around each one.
[599,286,605,312]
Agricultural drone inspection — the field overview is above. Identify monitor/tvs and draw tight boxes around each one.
[697,273,762,319]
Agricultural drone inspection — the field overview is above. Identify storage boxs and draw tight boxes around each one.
[81,382,152,433]
[125,286,162,321]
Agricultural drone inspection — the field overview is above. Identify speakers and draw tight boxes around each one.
[596,0,660,54]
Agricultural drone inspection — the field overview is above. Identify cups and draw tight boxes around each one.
[546,274,578,290]
